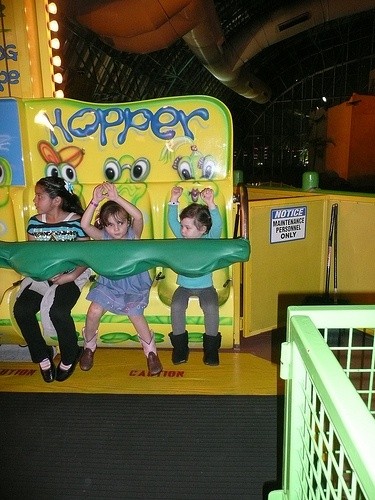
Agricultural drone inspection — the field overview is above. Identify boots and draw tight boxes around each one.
[168,330,190,365]
[137,330,163,375]
[80,327,99,372]
[203,333,222,365]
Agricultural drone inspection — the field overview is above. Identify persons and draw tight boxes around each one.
[80,182,163,375]
[13,176,90,383]
[168,187,222,366]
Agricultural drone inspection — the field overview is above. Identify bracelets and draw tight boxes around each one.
[90,200,99,207]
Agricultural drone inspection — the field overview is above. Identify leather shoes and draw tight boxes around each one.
[39,346,55,382]
[55,347,83,382]
